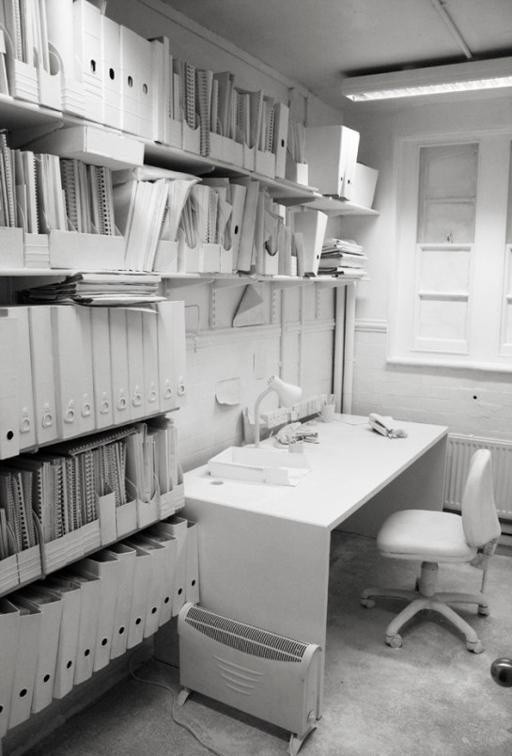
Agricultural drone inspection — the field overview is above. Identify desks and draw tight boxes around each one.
[178,414,453,725]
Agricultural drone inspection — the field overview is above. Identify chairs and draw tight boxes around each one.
[364,450,502,654]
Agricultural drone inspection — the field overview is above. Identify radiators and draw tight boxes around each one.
[445,434,512,523]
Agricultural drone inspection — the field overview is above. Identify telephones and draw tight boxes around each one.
[368,413,402,436]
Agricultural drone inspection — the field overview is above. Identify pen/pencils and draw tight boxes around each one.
[322,396,336,406]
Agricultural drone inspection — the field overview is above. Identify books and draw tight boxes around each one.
[0,415,181,561]
[148,37,202,130]
[204,63,379,281]
[0,128,116,235]
[207,445,321,488]
[1,0,51,75]
[113,178,203,272]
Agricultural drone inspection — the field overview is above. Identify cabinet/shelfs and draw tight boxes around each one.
[1,95,377,752]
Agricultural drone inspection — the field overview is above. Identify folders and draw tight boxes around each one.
[128,534,165,639]
[0,598,20,739]
[8,594,45,729]
[39,576,82,700]
[0,303,189,459]
[139,528,178,628]
[119,537,151,650]
[303,125,360,202]
[21,586,63,714]
[60,565,101,686]
[103,543,136,659]
[293,210,328,277]
[154,517,187,617]
[187,519,201,604]
[80,553,121,673]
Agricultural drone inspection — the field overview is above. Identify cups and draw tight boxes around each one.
[318,406,335,422]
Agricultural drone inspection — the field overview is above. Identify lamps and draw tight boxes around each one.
[341,55,512,102]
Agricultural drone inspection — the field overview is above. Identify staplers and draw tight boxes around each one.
[295,432,320,444]
